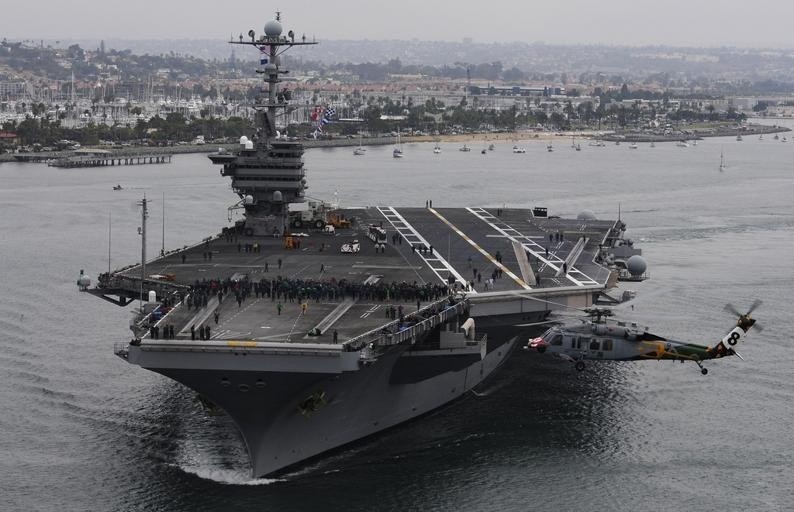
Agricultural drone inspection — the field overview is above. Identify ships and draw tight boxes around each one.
[73,11,771,477]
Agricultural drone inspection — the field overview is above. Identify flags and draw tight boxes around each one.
[310,105,325,121]
[310,108,336,140]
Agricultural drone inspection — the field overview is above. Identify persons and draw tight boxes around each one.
[560,234,563,241]
[174,226,284,276]
[425,199,428,207]
[429,200,432,208]
[548,234,553,242]
[318,263,326,273]
[562,261,566,273]
[536,275,540,286]
[500,209,503,216]
[582,234,585,242]
[320,241,325,249]
[392,231,433,255]
[496,209,500,215]
[546,249,548,255]
[554,233,558,241]
[465,249,504,276]
[374,242,379,253]
[527,251,529,259]
[380,242,385,253]
